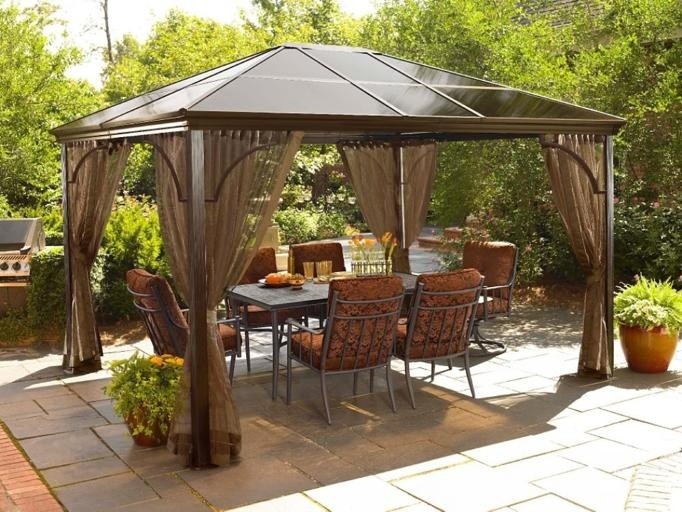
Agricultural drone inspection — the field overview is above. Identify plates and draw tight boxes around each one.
[258,279,288,288]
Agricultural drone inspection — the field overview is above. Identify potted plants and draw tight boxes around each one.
[613,271,682,373]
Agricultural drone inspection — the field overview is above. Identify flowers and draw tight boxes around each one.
[101,353,185,442]
[348,231,398,274]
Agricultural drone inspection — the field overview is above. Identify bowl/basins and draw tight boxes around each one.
[288,280,304,290]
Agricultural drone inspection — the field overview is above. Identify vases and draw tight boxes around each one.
[123,402,173,447]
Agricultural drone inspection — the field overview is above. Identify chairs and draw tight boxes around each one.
[223,247,302,372]
[461,240,520,357]
[286,274,406,425]
[288,243,347,329]
[369,268,486,409]
[126,269,244,385]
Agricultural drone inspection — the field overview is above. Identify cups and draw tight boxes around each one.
[316,262,327,282]
[323,260,332,278]
[302,262,314,283]
